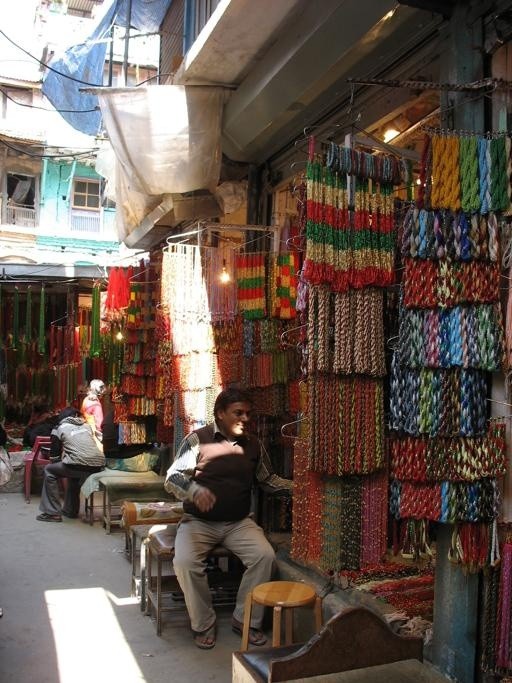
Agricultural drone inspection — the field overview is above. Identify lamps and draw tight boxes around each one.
[219,229,230,283]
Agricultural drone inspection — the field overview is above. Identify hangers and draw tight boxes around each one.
[162,239,199,258]
[165,226,246,252]
[231,231,299,254]
[50,266,160,372]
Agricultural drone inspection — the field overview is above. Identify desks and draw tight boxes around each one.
[98,476,179,535]
[120,502,183,564]
[144,524,240,636]
[80,467,159,526]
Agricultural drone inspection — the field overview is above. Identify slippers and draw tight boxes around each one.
[232,622,267,646]
[194,624,216,649]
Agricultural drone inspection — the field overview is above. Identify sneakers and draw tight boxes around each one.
[37,513,61,522]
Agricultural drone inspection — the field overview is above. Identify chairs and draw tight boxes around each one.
[23,436,67,504]
[231,606,424,683]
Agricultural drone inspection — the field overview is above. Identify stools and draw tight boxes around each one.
[130,523,179,612]
[240,580,323,652]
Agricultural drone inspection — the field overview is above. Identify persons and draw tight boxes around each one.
[36,406,107,522]
[164,389,293,650]
[84,379,104,441]
